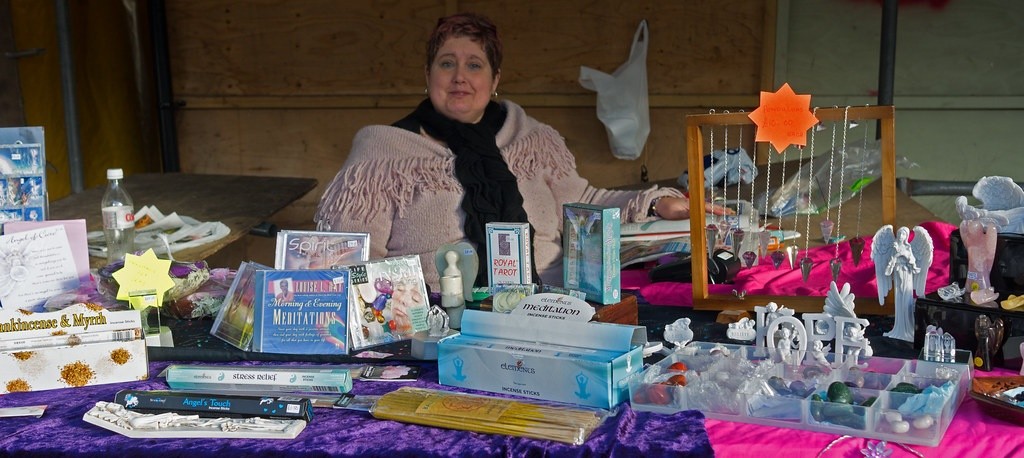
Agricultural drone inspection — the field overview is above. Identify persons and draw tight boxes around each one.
[314,11,734,299]
[778,327,800,360]
[276,279,294,304]
[846,327,862,365]
[812,340,832,370]
[882,226,921,342]
[286,234,318,271]
[766,303,784,332]
[729,319,755,329]
[382,275,426,332]
[965,222,991,293]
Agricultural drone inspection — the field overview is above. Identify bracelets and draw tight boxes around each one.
[648,196,672,217]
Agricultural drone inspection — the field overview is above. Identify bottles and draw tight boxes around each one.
[101,168,134,266]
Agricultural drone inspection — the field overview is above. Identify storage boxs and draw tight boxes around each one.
[274,230,370,269]
[561,202,621,303]
[629,340,972,448]
[911,291,1024,369]
[486,222,532,294]
[330,254,432,350]
[437,308,648,411]
[251,269,350,355]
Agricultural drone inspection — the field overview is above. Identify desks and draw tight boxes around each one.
[47,170,319,270]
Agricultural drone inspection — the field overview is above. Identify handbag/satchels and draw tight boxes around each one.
[578,19,651,161]
[678,145,760,195]
